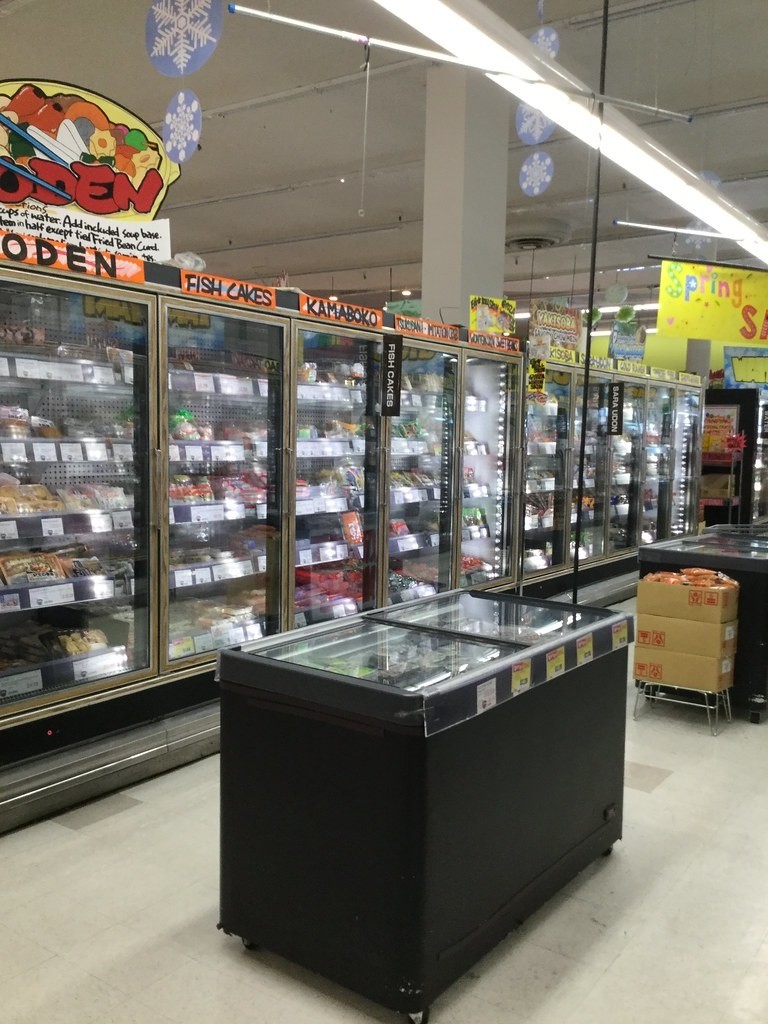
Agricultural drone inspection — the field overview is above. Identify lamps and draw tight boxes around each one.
[374,0,768,266]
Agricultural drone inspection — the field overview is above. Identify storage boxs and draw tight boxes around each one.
[632,578,740,693]
[699,474,736,498]
